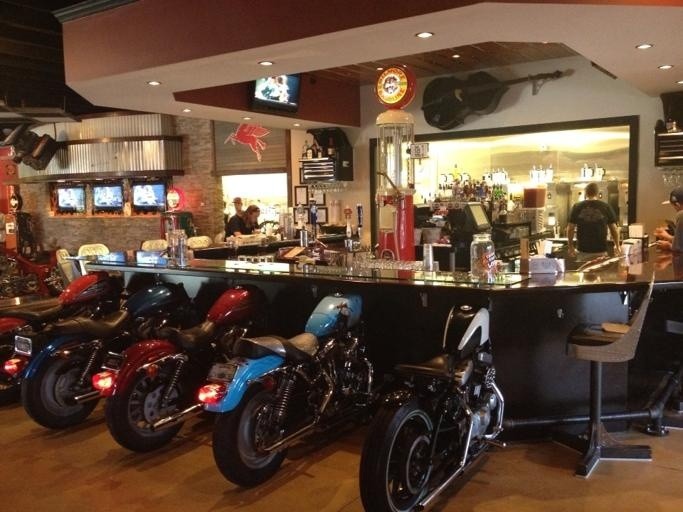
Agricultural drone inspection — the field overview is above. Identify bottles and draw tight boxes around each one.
[306,145,314,158]
[581,163,588,177]
[470,234,496,283]
[546,160,553,179]
[311,139,317,156]
[538,165,545,180]
[168,229,190,267]
[302,140,307,158]
[483,168,507,181]
[327,138,334,157]
[318,146,322,158]
[529,165,538,180]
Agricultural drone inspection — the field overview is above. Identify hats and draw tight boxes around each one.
[670,189,683,205]
[232,198,242,203]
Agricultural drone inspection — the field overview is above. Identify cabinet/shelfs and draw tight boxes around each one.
[653,90,683,166]
[299,128,353,184]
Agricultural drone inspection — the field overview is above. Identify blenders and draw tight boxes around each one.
[374,62,416,261]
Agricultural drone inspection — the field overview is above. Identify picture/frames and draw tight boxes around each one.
[293,186,328,224]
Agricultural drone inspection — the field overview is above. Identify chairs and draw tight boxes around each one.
[78,243,109,276]
[550,271,655,479]
[186,236,212,249]
[141,239,168,253]
[56,248,81,289]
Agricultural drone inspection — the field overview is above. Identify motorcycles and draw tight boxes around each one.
[77,284,262,453]
[197,296,374,489]
[357,304,503,512]
[6,285,183,431]
[0,271,110,409]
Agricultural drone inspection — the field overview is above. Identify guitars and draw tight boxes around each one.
[420,71,562,130]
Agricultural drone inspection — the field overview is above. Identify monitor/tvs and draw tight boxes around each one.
[131,183,166,212]
[92,185,124,209]
[251,72,303,111]
[467,203,494,231]
[57,186,86,211]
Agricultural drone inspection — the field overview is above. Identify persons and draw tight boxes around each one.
[225,206,260,241]
[567,183,621,263]
[653,188,683,253]
[232,197,244,216]
